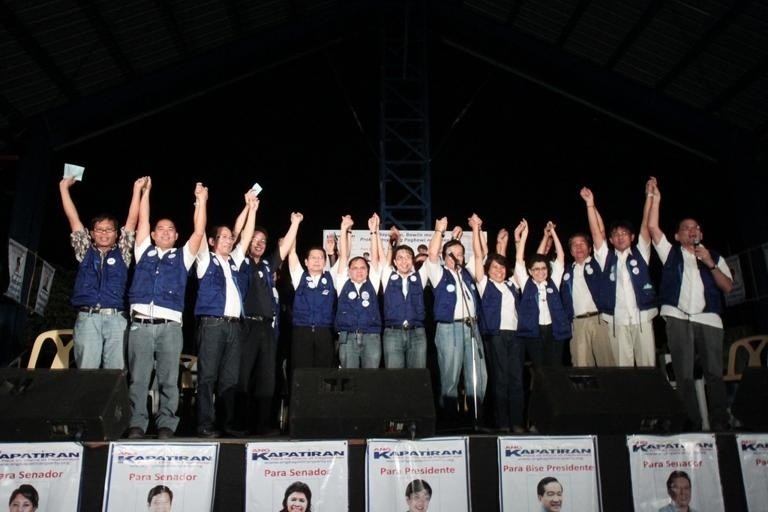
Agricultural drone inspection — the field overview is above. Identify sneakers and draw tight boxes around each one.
[713,419,733,432]
[157,427,174,439]
[529,425,538,433]
[128,427,146,439]
[224,427,233,432]
[500,425,524,433]
[688,422,702,431]
[200,423,212,434]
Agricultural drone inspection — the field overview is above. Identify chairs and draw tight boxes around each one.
[28,327,76,436]
[279,358,345,437]
[658,353,677,388]
[722,334,768,432]
[178,352,198,388]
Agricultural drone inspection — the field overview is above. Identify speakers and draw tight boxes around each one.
[531,364,685,435]
[729,364,768,429]
[0,370,134,441]
[285,366,439,437]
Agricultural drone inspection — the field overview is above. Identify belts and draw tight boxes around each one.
[79,307,122,314]
[388,324,418,331]
[133,317,171,324]
[576,311,599,319]
[439,319,472,325]
[202,314,240,322]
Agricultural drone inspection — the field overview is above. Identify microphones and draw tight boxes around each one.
[447,250,463,268]
[693,238,700,258]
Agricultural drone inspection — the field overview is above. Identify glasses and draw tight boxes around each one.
[95,227,116,233]
[612,232,628,238]
[217,235,235,241]
[682,226,700,232]
[253,238,267,243]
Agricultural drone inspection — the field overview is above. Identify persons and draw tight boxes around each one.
[374,176,734,436]
[280,481,312,512]
[340,213,383,369]
[362,252,371,262]
[232,189,303,437]
[289,211,352,367]
[537,476,563,512]
[658,471,699,512]
[147,485,173,512]
[192,182,261,438]
[60,175,148,370]
[127,176,209,439]
[8,484,38,512]
[405,479,432,512]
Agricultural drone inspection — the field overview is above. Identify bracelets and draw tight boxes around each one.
[369,231,376,234]
[192,202,200,206]
[347,230,352,233]
